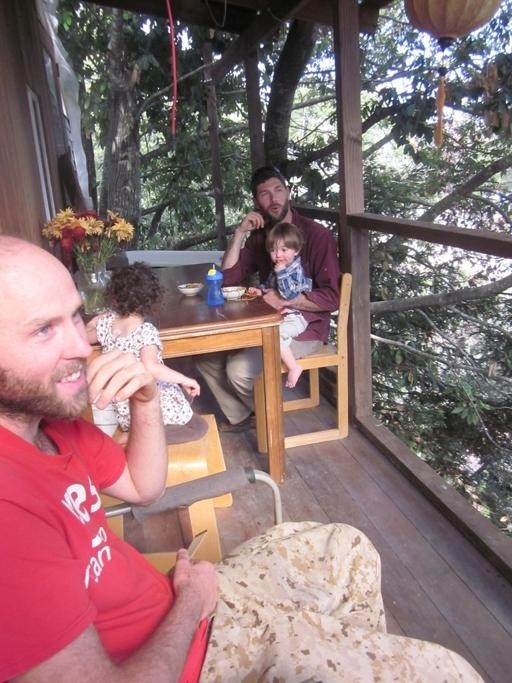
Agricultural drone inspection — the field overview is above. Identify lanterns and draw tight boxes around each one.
[402,0,502,146]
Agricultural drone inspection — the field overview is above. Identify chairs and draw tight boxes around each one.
[86,465,287,551]
[247,267,355,456]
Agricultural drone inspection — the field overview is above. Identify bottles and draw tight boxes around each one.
[205,263,225,306]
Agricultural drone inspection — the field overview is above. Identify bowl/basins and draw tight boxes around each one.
[221,286,246,300]
[177,283,204,296]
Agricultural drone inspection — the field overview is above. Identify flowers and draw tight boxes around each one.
[42,204,137,250]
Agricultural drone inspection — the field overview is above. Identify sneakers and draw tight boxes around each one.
[219,416,252,432]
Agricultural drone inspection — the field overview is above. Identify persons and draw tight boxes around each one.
[0,231,486,682]
[191,164,341,433]
[84,258,210,448]
[248,221,313,388]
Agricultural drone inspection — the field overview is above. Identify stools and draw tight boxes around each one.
[93,412,236,568]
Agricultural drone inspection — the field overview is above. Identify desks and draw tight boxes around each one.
[90,263,290,485]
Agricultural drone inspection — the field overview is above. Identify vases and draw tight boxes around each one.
[66,248,110,321]
